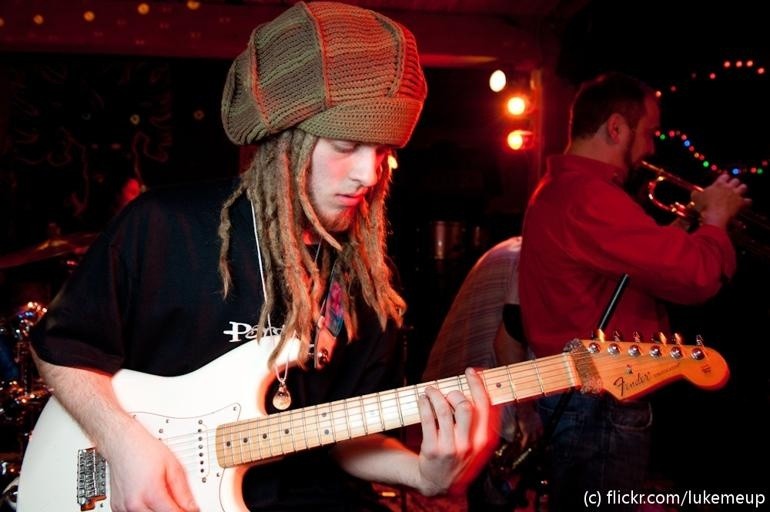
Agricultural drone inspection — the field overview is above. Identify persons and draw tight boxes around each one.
[30,1,502,511]
[412,234,547,509]
[522,67,753,506]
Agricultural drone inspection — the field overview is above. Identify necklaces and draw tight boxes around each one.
[245,188,327,412]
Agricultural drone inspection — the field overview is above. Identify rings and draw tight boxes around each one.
[455,400,470,410]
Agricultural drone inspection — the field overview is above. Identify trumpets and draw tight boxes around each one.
[639,158,767,248]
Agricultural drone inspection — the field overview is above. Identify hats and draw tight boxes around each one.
[217,0,432,149]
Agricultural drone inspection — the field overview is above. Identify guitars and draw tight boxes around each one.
[15,333,731,512]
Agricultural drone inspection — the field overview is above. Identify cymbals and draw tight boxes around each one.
[2,233,97,269]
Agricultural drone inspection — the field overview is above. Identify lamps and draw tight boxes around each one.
[507,129,533,151]
[507,93,531,117]
